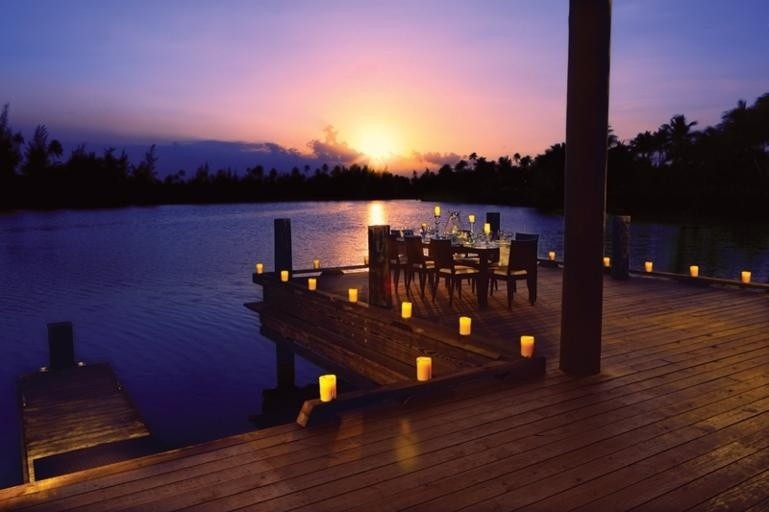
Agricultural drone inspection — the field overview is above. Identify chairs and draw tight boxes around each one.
[389,229,540,309]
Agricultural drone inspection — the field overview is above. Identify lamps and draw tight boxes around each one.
[417,356,431,383]
[257,263,264,273]
[313,256,320,269]
[459,317,472,336]
[318,374,338,401]
[402,302,412,318]
[521,336,533,361]
[309,278,316,291]
[281,270,289,282]
[364,256,369,266]
[348,290,358,303]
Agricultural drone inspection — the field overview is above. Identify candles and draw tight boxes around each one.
[546,250,751,287]
[469,215,475,222]
[434,206,440,216]
[484,222,490,234]
